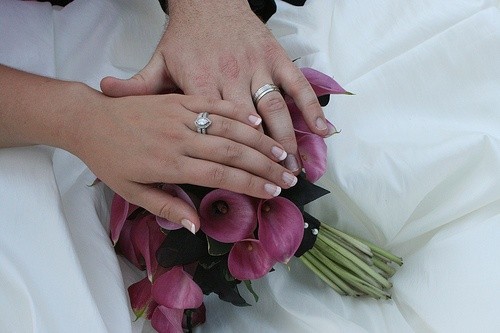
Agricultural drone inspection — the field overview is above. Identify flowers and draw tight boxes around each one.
[85,56,404,333]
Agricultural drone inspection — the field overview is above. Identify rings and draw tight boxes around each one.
[194,112,212,136]
[253,84,280,108]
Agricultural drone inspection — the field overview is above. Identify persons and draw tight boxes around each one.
[1,63,298,235]
[20,1,328,178]
